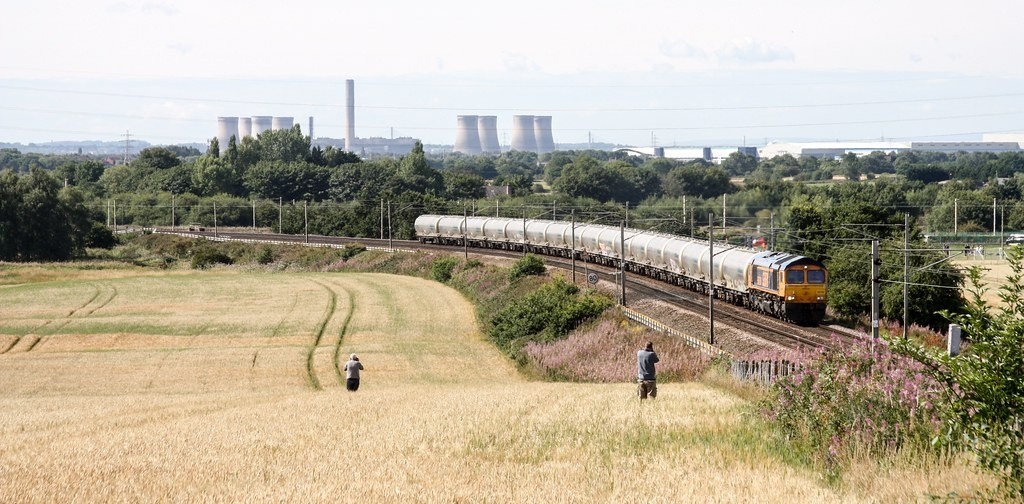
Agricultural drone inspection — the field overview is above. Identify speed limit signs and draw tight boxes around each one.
[587,272,599,284]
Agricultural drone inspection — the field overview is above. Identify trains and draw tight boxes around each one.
[413,214,829,327]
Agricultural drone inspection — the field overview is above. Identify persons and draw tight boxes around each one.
[945,244,949,254]
[964,244,970,256]
[344,353,363,391]
[637,341,659,399]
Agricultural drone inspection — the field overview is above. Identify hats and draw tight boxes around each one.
[350,353,357,360]
